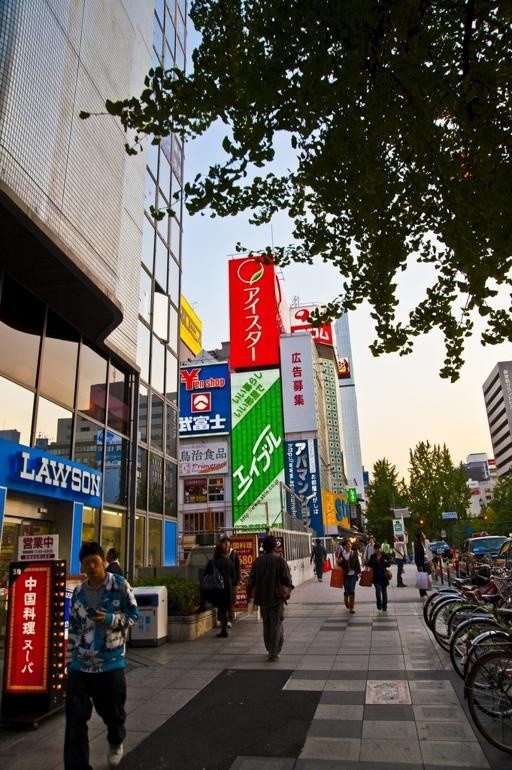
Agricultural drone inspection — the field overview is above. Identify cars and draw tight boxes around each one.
[497,536,512,575]
[427,539,454,561]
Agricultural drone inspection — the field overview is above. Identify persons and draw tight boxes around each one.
[310,539,327,582]
[64,542,140,770]
[105,549,122,576]
[335,530,433,613]
[195,544,236,637]
[217,537,241,628]
[247,535,294,661]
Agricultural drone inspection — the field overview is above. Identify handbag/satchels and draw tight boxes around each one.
[359,570,372,587]
[387,571,392,579]
[330,568,343,588]
[403,554,407,562]
[202,574,224,590]
[323,558,332,572]
[281,583,291,600]
[414,571,428,589]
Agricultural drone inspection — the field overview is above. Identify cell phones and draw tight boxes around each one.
[86,608,97,616]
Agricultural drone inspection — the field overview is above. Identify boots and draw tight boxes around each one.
[344,592,355,613]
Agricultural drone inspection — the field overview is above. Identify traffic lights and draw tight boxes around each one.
[418,519,424,525]
[483,515,488,521]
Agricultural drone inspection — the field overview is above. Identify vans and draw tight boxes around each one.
[458,531,507,586]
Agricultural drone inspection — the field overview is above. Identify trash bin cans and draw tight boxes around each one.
[123,586,168,647]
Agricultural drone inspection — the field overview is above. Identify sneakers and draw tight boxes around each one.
[376,602,387,612]
[216,620,232,637]
[107,743,124,766]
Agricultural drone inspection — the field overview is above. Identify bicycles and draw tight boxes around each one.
[422,553,512,755]
